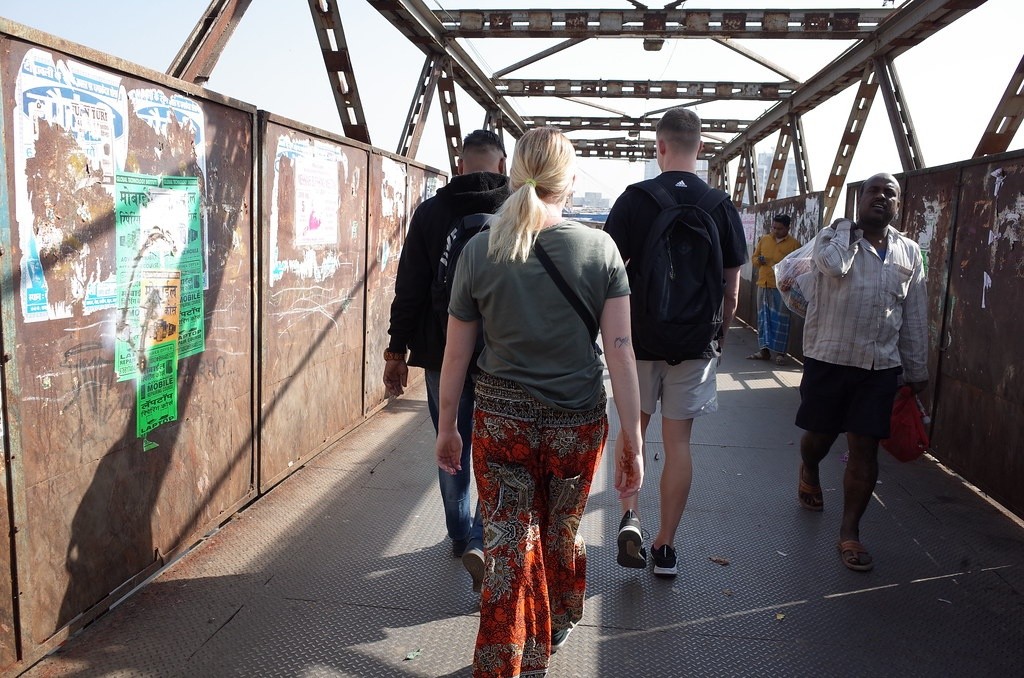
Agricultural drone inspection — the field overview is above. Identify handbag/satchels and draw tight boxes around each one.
[881,382,930,463]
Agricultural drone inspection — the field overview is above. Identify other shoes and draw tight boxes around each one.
[452,516,474,557]
[462,548,485,594]
[550,620,581,651]
[745,352,771,360]
[777,355,786,365]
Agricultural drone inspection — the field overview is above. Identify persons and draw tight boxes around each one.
[385,129,509,591]
[795,173,930,571]
[745,214,802,366]
[435,124,644,678]
[603,107,749,575]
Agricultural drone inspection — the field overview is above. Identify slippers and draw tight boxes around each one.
[799,462,823,512]
[837,538,873,571]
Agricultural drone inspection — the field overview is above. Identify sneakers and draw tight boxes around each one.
[650,542,679,575]
[617,509,650,569]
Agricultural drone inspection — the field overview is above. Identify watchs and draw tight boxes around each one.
[384,348,405,361]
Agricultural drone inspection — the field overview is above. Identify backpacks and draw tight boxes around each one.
[626,180,731,359]
[437,213,501,301]
